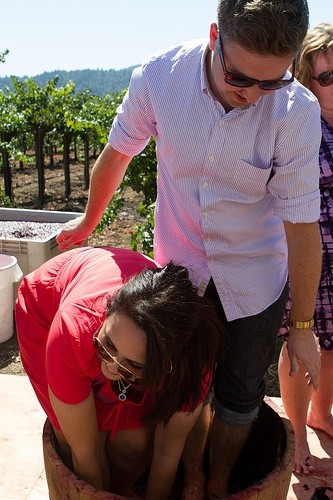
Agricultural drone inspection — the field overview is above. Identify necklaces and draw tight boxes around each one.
[116,376,133,401]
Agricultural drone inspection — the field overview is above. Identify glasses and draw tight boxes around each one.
[216,29,297,90]
[93,322,145,384]
[312,69,333,87]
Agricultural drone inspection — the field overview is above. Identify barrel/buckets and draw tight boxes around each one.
[0,254,24,344]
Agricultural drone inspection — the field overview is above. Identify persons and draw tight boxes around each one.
[14,243,225,500]
[55,0,324,500]
[273,22,333,474]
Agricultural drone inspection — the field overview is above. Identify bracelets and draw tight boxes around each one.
[288,317,316,330]
